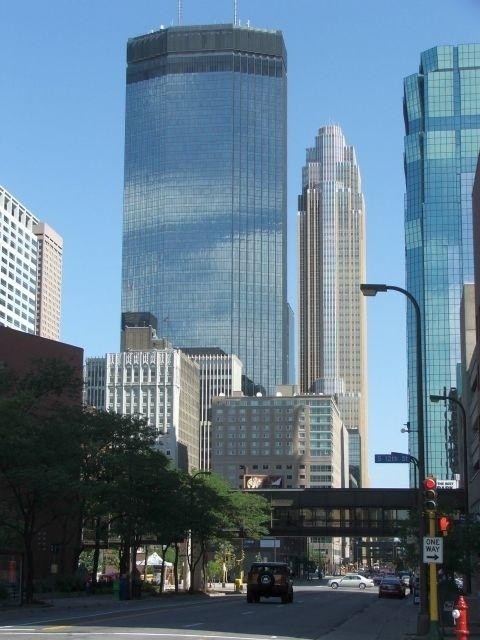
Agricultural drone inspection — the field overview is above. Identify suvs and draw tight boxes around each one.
[248,562,293,604]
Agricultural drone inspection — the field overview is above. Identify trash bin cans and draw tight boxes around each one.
[437,569,460,627]
[119,572,129,601]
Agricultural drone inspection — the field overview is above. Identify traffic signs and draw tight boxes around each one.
[375,453,410,463]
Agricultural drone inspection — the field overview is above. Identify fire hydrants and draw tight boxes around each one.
[452,594,471,640]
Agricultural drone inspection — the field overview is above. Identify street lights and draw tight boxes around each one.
[191,471,212,491]
[430,395,472,594]
[361,284,429,540]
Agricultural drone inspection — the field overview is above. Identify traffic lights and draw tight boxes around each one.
[439,517,450,531]
[426,478,437,510]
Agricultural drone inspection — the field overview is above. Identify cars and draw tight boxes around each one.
[409,567,449,604]
[328,575,374,589]
[379,577,405,599]
[363,570,395,584]
